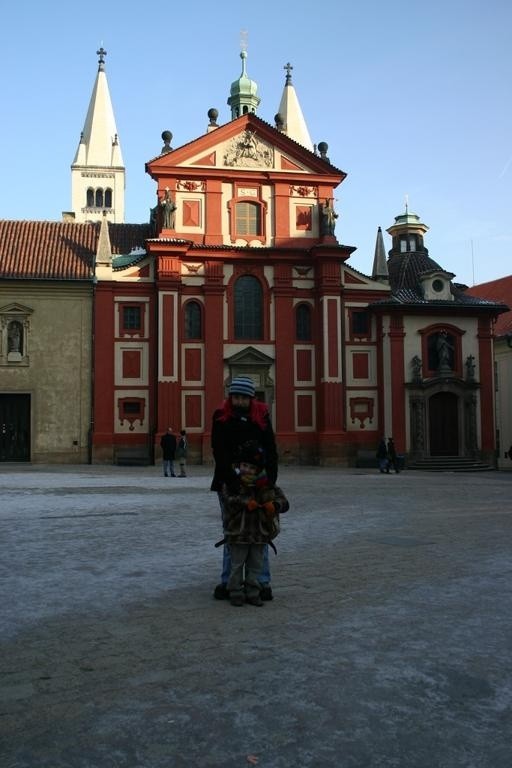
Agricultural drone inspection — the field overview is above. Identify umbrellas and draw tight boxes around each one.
[164,473,187,477]
[214,582,273,607]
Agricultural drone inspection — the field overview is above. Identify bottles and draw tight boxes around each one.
[237,444,264,464]
[229,377,255,397]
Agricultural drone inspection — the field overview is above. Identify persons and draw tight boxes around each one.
[322,200,338,235]
[377,437,400,474]
[176,430,187,477]
[9,324,21,352]
[160,192,175,229]
[210,376,290,607]
[160,428,176,477]
[241,130,257,160]
